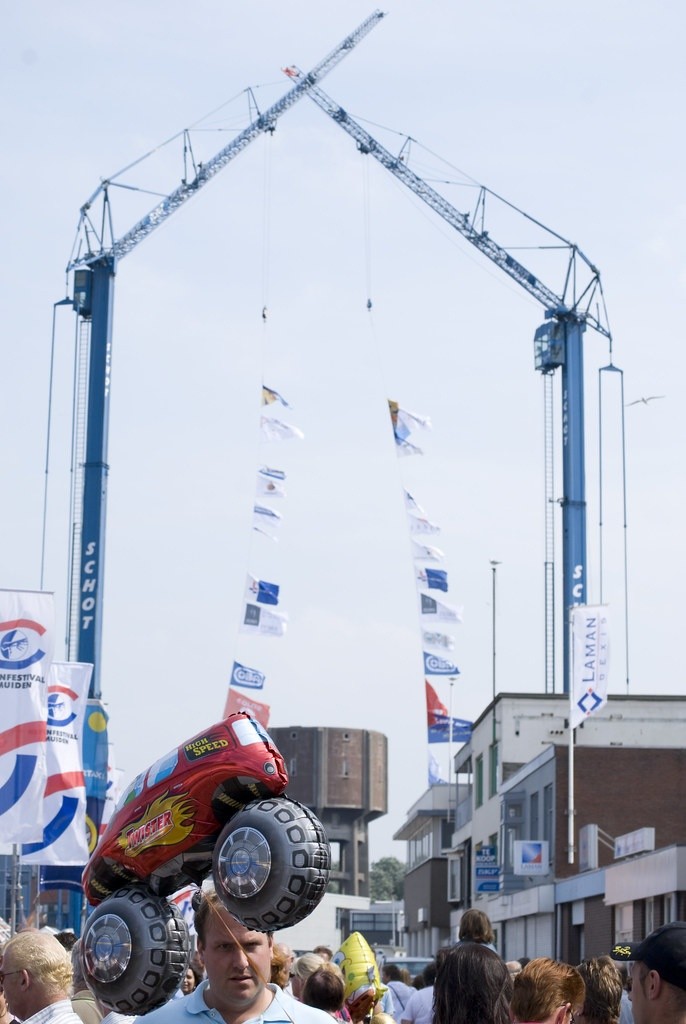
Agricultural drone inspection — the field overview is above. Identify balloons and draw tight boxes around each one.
[80,711,331,1016]
[329,931,389,1024]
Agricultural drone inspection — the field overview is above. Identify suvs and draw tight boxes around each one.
[379,956,436,981]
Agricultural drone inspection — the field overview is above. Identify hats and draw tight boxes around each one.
[609,921,686,992]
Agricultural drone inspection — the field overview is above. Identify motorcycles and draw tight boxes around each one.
[79,711,331,1016]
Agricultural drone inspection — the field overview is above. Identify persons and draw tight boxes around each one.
[0,891,686,1024]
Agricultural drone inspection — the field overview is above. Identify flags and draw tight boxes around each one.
[223,394,307,733]
[388,403,472,788]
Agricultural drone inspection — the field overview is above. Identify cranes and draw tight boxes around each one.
[282,64,630,697]
[38,9,386,835]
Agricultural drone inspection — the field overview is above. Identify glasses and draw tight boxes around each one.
[561,1005,575,1024]
[0,969,29,984]
